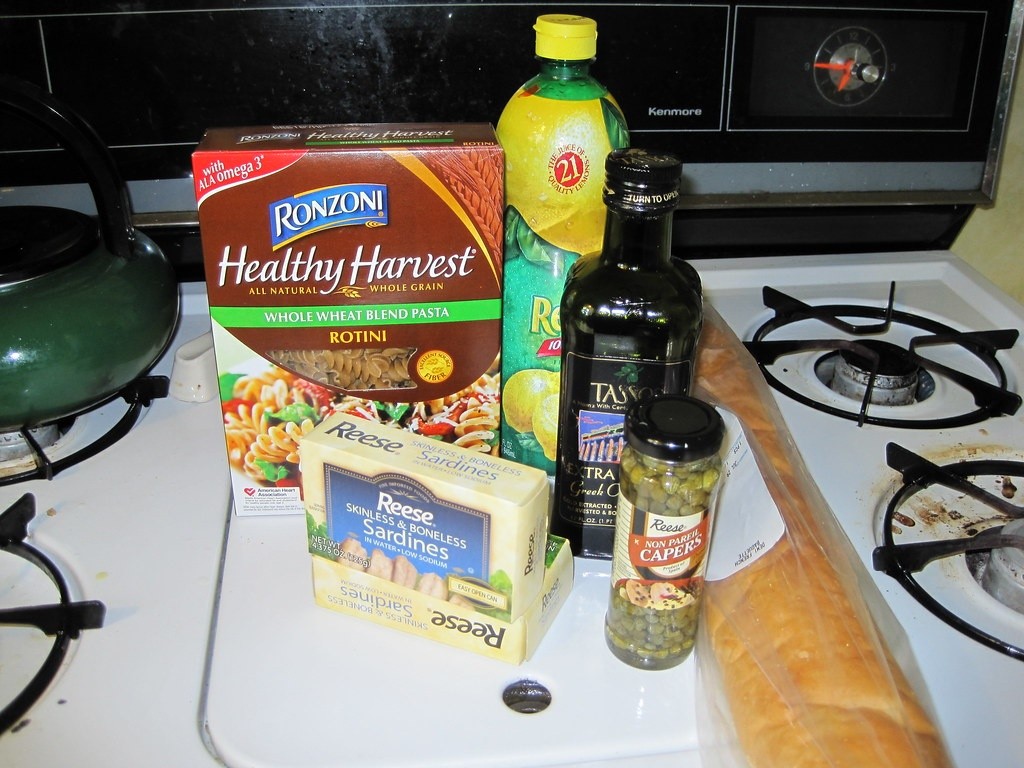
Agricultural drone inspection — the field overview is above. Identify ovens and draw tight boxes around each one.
[727,0,1023,160]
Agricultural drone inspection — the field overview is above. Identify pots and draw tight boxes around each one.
[0,203,182,427]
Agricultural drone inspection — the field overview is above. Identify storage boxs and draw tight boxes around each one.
[299,411,574,664]
[189,116,505,517]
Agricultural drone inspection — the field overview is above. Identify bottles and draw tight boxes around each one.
[497,13,628,471]
[548,148,706,559]
[604,397,726,671]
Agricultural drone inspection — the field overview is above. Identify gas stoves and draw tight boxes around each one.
[0,204,182,768]
[656,236,1024,768]
